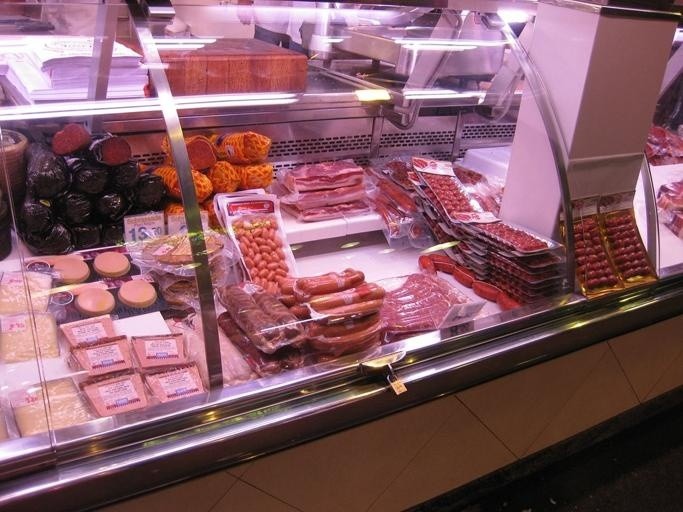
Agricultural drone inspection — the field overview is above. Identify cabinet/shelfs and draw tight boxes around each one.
[0,1,683,512]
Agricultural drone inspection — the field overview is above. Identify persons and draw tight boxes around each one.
[237,0,316,52]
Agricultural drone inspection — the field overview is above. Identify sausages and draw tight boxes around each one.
[217,172,651,368]
